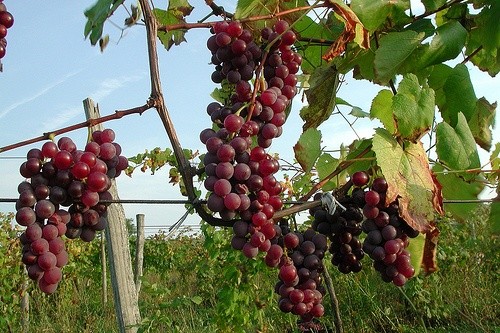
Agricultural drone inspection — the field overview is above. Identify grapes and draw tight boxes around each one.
[0,0,14,72]
[16,124,130,296]
[199,15,419,316]
